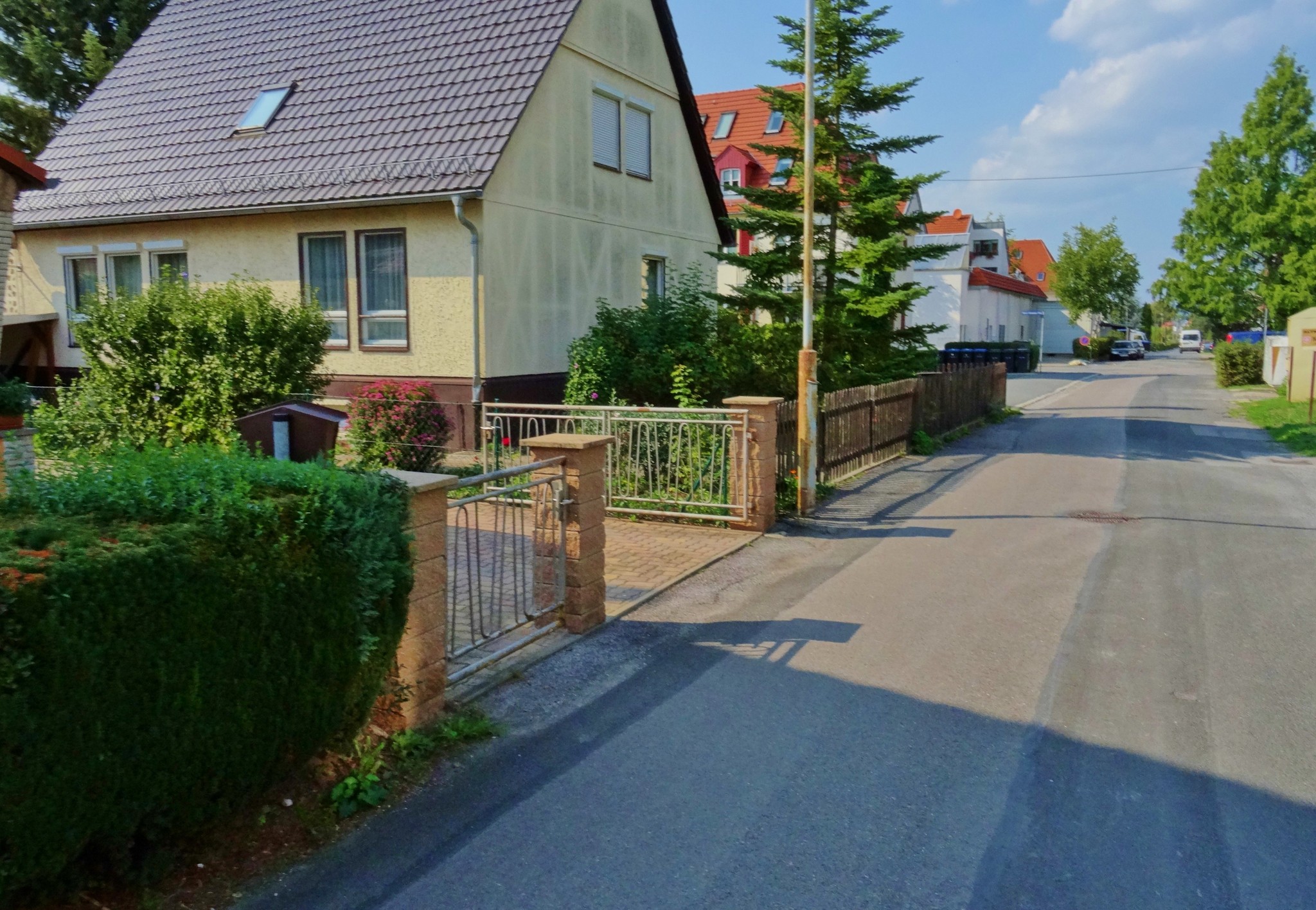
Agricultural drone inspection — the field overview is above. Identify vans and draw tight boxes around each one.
[1179,330,1201,354]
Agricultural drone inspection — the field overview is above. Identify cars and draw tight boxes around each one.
[1109,340,1145,361]
